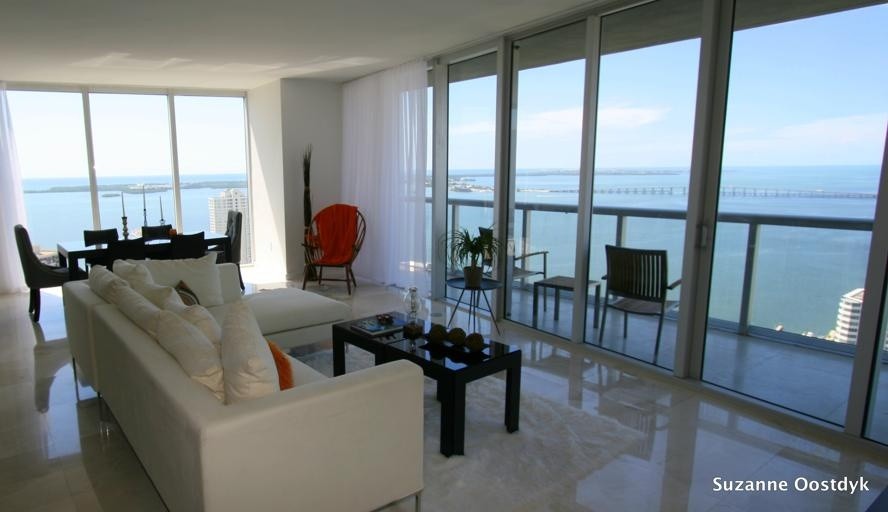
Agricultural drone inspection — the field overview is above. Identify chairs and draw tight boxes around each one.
[474,249,550,315]
[302,204,370,295]
[13,223,85,323]
[106,236,146,270]
[83,228,119,272]
[596,243,684,365]
[171,231,206,262]
[209,209,244,293]
[141,225,175,239]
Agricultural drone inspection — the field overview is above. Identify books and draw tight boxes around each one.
[351,318,404,336]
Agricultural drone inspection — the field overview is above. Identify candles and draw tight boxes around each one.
[158,196,163,219]
[141,185,146,209]
[120,191,125,217]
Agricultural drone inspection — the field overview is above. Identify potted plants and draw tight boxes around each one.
[438,221,502,290]
[301,142,319,282]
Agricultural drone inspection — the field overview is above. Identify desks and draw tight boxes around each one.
[531,274,603,333]
[446,275,502,338]
[56,232,231,273]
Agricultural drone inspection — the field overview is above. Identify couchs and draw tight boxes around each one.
[60,261,426,510]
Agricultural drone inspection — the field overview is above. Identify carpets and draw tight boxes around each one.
[291,342,649,512]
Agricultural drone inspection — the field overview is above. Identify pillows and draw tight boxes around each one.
[218,298,281,406]
[180,305,222,347]
[138,280,188,313]
[109,258,154,288]
[125,251,225,308]
[263,338,292,391]
[173,278,200,308]
